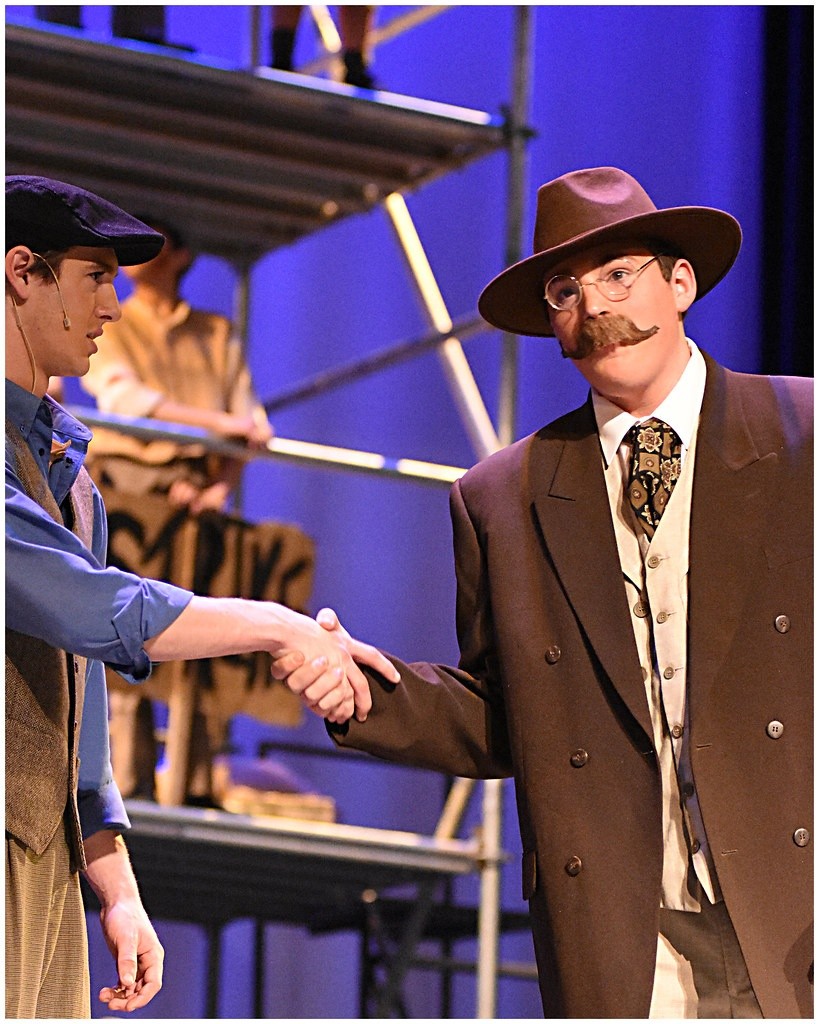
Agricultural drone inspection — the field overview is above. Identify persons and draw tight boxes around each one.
[35,4,380,89]
[6,174,401,1019]
[267,205,813,1019]
[78,224,317,804]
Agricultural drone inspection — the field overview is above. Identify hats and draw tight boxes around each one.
[477,166,744,337]
[5,174,165,267]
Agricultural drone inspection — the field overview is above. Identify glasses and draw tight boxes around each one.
[538,251,666,312]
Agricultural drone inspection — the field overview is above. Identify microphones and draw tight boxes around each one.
[33,254,71,328]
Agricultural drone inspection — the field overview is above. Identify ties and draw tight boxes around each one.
[621,418,683,543]
